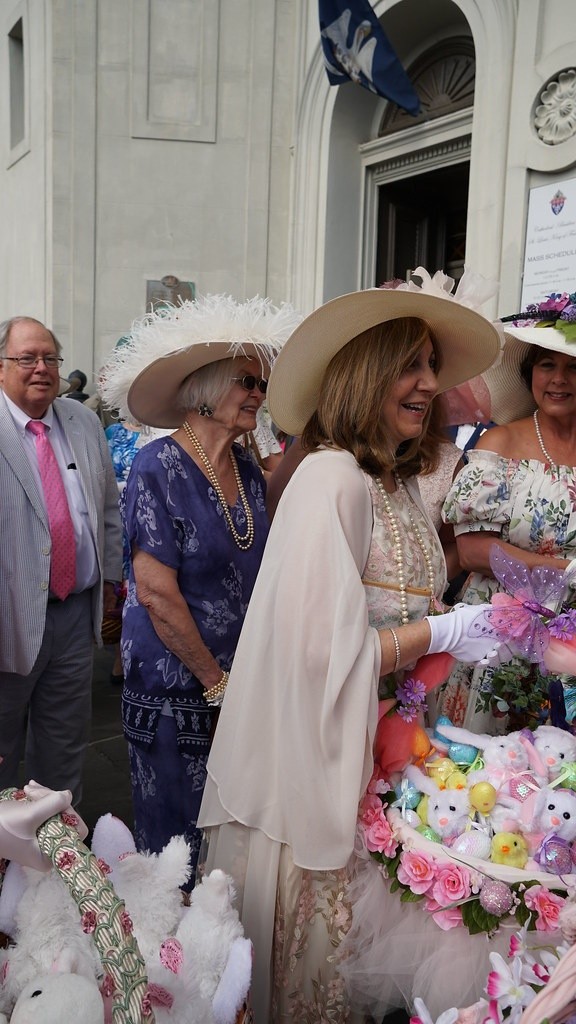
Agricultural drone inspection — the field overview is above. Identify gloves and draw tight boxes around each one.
[0,780,89,873]
[425,601,523,669]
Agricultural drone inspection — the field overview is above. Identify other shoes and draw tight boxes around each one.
[111,654,125,686]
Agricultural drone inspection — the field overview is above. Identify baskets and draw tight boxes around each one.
[519,942,576,1024]
[1,784,255,1024]
[359,603,576,931]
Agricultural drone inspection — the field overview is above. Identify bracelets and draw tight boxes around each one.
[389,628,400,673]
[202,671,228,700]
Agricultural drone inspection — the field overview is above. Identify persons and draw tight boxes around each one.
[427,293,576,737]
[134,415,284,482]
[66,366,141,685]
[99,301,311,901]
[417,440,466,582]
[195,276,508,1024]
[1,317,123,817]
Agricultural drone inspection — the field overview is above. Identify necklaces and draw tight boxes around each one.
[376,474,435,624]
[533,409,555,467]
[183,421,254,549]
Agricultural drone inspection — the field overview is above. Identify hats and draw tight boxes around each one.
[467,289,576,428]
[266,266,505,438]
[98,294,309,430]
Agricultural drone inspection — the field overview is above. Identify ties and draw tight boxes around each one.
[25,420,77,601]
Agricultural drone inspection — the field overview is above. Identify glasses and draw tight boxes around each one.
[0,351,64,370]
[229,372,269,395]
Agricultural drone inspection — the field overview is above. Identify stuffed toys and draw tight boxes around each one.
[0,814,251,1024]
[392,725,576,874]
